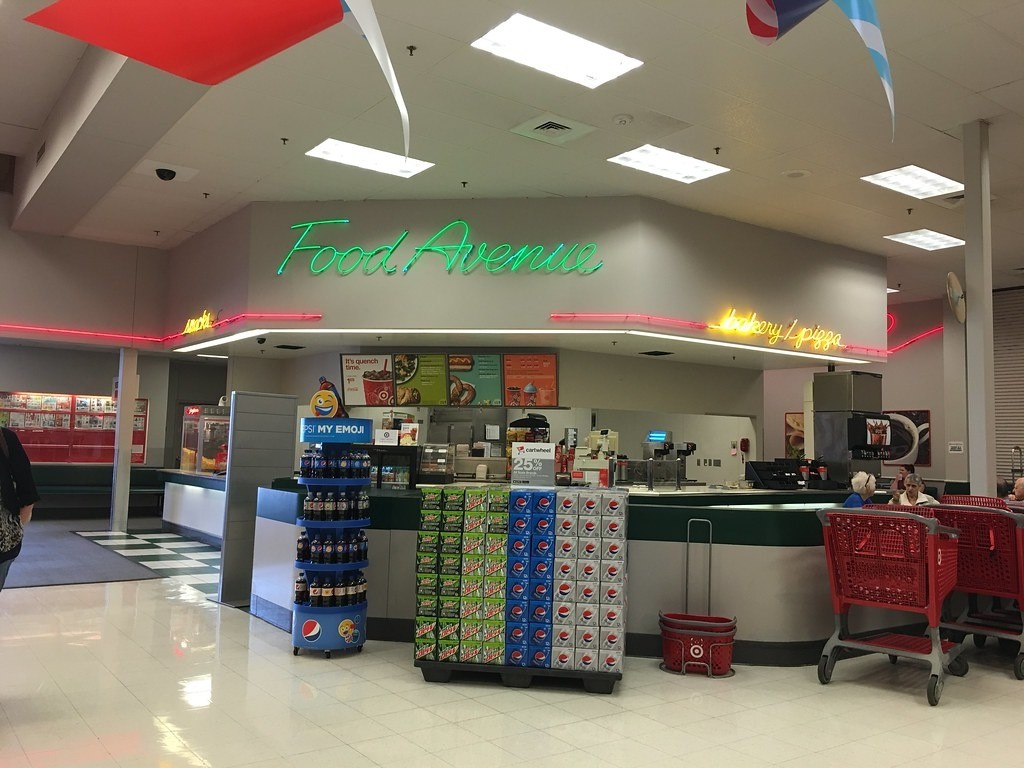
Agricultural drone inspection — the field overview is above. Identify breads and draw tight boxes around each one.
[449,375,476,405]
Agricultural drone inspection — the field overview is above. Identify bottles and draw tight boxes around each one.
[295,451,371,604]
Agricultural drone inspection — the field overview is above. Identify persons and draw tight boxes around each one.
[0,426,39,593]
[842,471,876,507]
[890,464,926,493]
[1007,477,1024,501]
[887,473,941,505]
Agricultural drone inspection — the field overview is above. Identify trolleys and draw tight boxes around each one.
[816,506,969,703]
[898,497,1024,678]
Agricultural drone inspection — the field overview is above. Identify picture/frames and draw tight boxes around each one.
[338,352,560,409]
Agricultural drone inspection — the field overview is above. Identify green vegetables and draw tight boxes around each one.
[395,354,417,381]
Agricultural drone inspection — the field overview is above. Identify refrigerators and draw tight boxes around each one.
[337,444,422,488]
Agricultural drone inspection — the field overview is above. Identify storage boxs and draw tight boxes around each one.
[412,487,628,673]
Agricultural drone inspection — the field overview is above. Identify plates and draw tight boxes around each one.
[395,355,418,384]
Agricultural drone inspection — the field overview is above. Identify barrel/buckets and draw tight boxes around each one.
[614,461,628,481]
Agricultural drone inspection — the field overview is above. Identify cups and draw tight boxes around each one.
[523,383,538,406]
[884,413,930,464]
[871,433,886,445]
[506,387,521,406]
[362,371,394,406]
[539,388,554,406]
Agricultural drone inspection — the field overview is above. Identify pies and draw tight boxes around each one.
[396,387,420,404]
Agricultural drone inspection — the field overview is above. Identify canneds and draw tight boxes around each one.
[371,472,395,482]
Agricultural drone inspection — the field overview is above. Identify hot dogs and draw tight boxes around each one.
[449,355,474,371]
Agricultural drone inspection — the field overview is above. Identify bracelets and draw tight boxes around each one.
[892,498,899,502]
